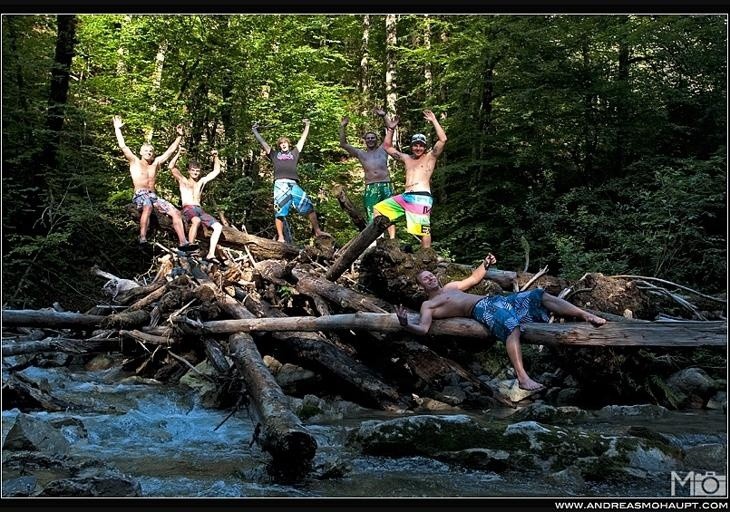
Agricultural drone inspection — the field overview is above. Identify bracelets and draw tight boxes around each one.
[400,322,408,327]
[177,134,183,137]
[387,127,394,131]
[382,113,386,118]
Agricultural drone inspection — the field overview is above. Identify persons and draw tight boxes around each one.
[372,109,447,248]
[168,147,223,264]
[251,117,331,244]
[112,115,200,252]
[340,108,396,240]
[393,252,608,391]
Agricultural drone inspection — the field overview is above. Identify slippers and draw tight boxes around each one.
[205,257,221,265]
[177,242,200,252]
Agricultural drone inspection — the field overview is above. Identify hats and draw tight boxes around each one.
[411,133,426,145]
